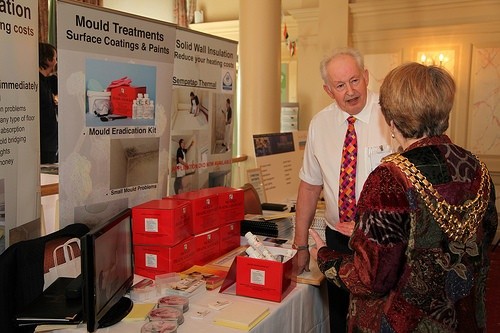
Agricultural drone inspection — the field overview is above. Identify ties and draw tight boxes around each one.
[338,117,358,223]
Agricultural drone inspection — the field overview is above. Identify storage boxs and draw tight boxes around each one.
[219,246,298,303]
[112,87,146,117]
[132,187,245,280]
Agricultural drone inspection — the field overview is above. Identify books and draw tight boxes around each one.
[240,214,293,239]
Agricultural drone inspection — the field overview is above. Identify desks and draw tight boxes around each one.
[35,209,331,333]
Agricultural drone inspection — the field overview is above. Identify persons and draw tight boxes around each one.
[38,40,60,165]
[292,52,398,333]
[189,91,201,117]
[221,98,232,152]
[173,138,194,195]
[308,60,500,333]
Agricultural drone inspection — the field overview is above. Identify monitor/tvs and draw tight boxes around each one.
[80,208,134,333]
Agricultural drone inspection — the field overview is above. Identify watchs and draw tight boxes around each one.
[290,242,309,255]
